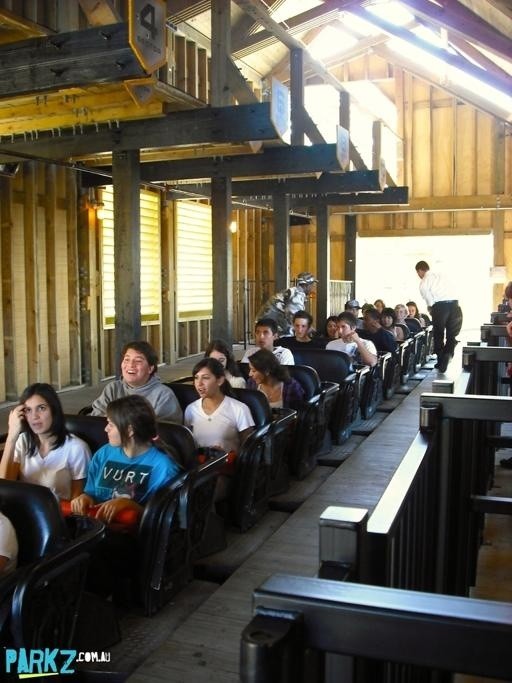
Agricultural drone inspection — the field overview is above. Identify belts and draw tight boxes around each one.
[435,300,458,304]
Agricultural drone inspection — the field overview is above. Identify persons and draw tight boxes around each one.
[499,281,512,467]
[0,270,433,666]
[415,261,463,369]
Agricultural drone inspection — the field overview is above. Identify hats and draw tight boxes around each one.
[345,300,363,310]
[296,271,320,285]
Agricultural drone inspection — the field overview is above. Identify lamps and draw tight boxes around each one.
[78,193,107,220]
